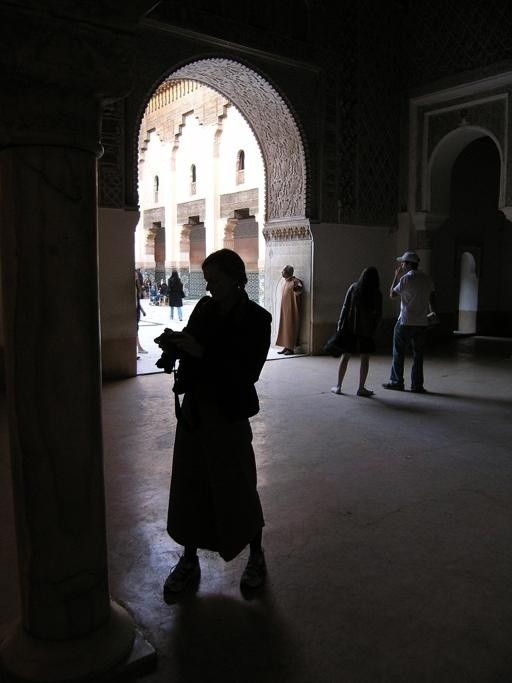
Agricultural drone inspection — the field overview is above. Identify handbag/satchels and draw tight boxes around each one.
[324,331,353,358]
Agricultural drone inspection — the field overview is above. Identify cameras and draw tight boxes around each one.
[155,327,180,374]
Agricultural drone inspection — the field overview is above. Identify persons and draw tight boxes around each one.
[154,246,273,606]
[381,251,439,392]
[330,265,384,397]
[274,263,305,355]
[131,265,187,361]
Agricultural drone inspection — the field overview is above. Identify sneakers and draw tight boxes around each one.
[284,349,294,355]
[164,554,201,595]
[277,348,287,354]
[330,387,341,394]
[411,387,425,392]
[383,382,404,390]
[358,389,373,396]
[240,552,268,586]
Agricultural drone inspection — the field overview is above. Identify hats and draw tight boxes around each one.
[397,252,421,263]
